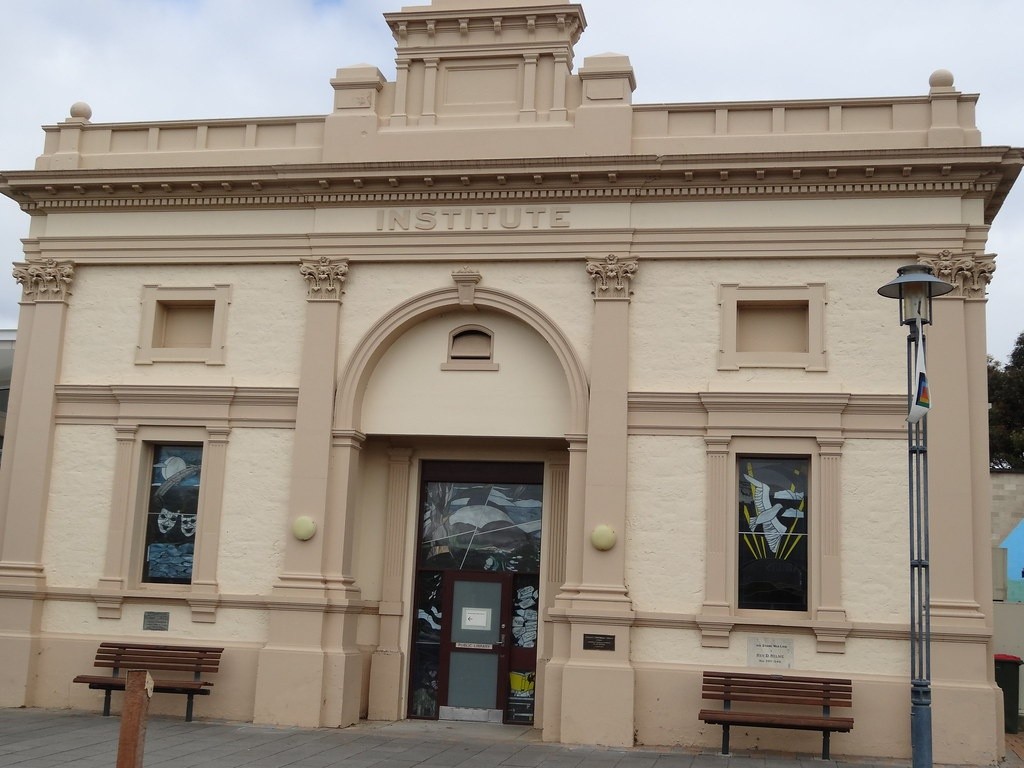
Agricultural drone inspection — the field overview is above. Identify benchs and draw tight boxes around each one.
[698,670,855,760]
[73,643,224,722]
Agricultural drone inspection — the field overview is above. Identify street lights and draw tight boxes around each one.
[877,265,957,768]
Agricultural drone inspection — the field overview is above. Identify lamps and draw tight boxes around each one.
[292,517,315,539]
[591,525,617,550]
